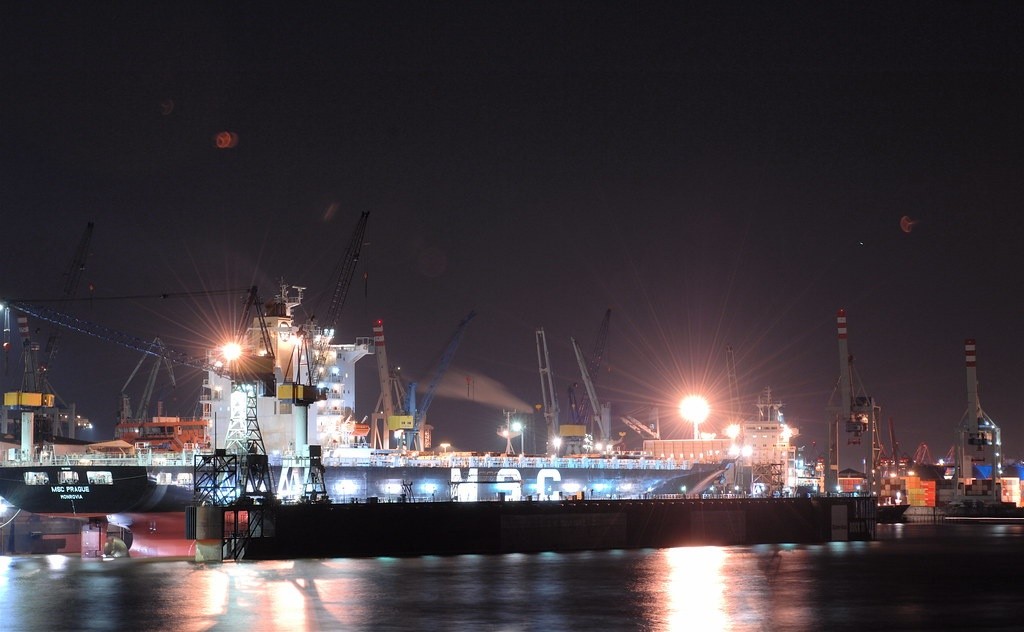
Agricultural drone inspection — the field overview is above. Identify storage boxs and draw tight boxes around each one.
[560,425,586,437]
[388,416,414,430]
[4,392,42,406]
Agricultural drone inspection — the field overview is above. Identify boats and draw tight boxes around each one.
[876,504,912,524]
[0,275,736,517]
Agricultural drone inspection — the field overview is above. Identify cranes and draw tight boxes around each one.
[0,202,370,504]
